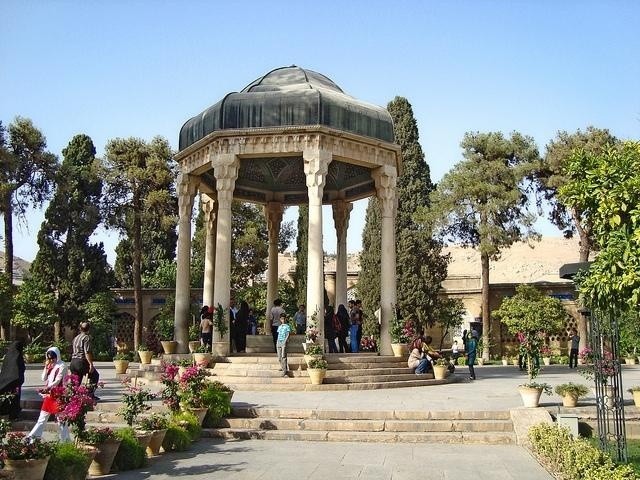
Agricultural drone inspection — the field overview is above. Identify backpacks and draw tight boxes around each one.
[332,315,342,333]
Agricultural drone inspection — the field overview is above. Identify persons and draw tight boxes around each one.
[268,297,306,377]
[324,297,370,353]
[198,305,213,352]
[462,329,479,379]
[452,341,459,366]
[230,297,256,353]
[408,336,441,374]
[21,346,70,445]
[70,321,101,405]
[568,329,580,368]
[517,327,540,375]
[0,340,26,422]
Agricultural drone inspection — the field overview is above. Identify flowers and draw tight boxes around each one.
[0,358,235,480]
[575,347,622,385]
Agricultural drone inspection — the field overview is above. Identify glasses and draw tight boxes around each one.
[48,357,54,359]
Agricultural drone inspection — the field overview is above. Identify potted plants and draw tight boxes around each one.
[194,344,212,364]
[112,352,130,375]
[301,305,329,386]
[625,384,640,408]
[386,302,450,382]
[490,284,569,409]
[186,321,201,353]
[457,353,640,367]
[135,343,156,364]
[211,303,232,357]
[553,381,591,408]
[158,333,178,354]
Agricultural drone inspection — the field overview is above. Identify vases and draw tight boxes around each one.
[590,384,619,410]
